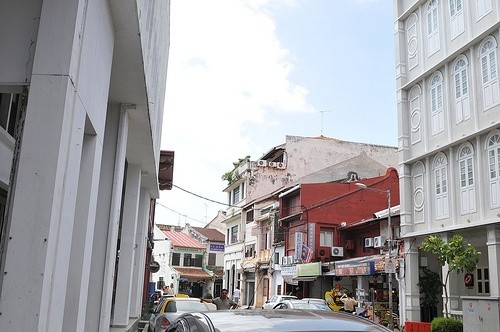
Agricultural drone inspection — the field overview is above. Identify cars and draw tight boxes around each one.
[163,310,396,332]
[273,301,333,311]
[261,295,299,311]
[302,298,325,301]
[155,298,211,332]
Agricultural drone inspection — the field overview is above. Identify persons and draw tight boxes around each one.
[201,289,237,310]
[203,289,212,299]
[162,286,174,295]
[340,293,357,312]
[232,287,240,308]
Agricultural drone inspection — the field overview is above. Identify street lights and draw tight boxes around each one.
[354,181,394,332]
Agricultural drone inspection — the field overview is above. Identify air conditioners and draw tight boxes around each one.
[331,246,344,256]
[365,237,374,247]
[269,162,277,167]
[276,162,285,169]
[283,255,298,265]
[318,248,330,258]
[373,237,385,249]
[257,160,267,167]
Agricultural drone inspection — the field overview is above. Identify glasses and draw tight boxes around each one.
[221,293,227,294]
